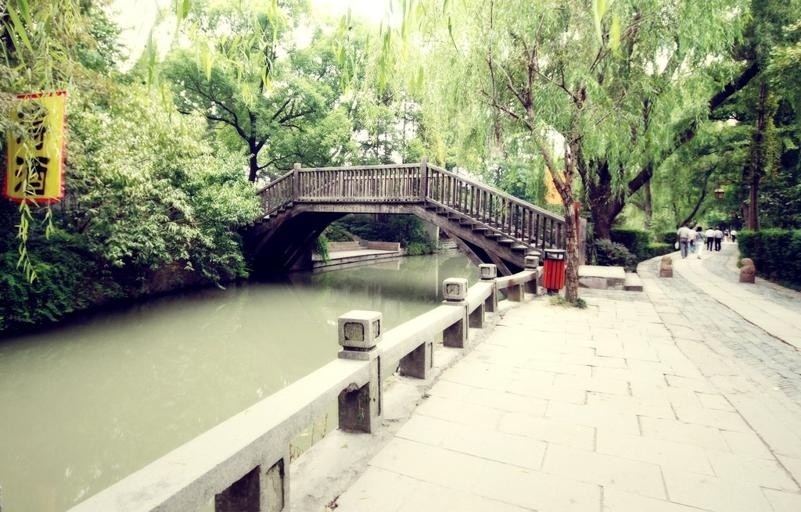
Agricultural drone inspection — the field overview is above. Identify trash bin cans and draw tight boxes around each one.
[542,249,567,296]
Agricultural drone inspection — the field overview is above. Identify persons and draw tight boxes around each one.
[676,223,736,259]
